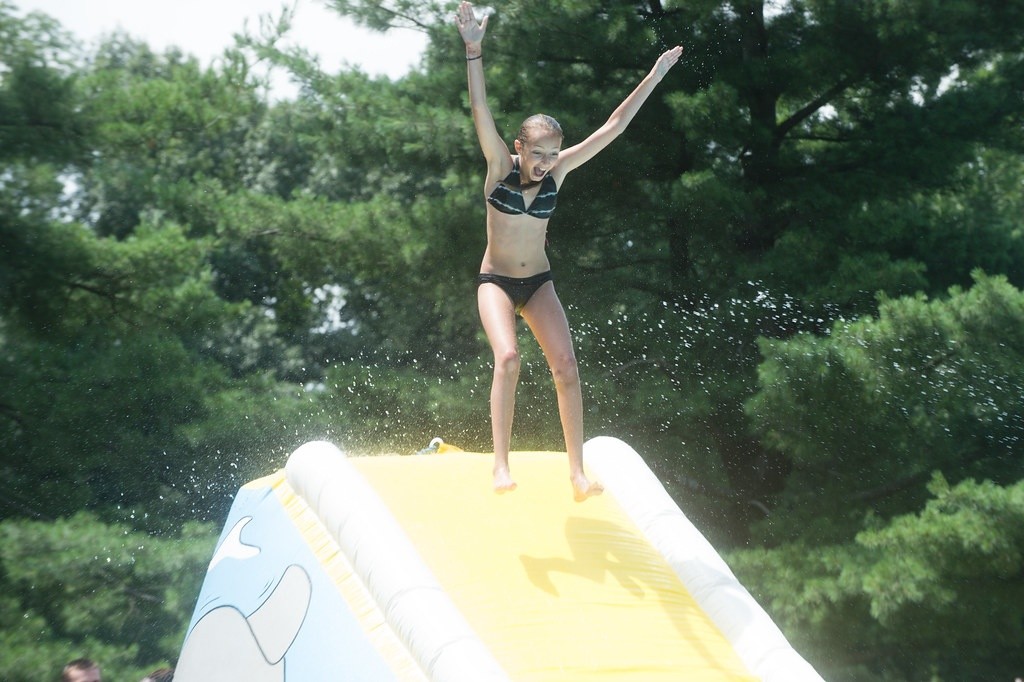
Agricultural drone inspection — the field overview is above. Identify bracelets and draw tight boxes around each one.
[465,54,482,61]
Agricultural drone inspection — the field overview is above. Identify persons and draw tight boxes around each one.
[454,1,687,504]
[58,651,177,682]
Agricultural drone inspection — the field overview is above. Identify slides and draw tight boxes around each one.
[350,446,758,682]
